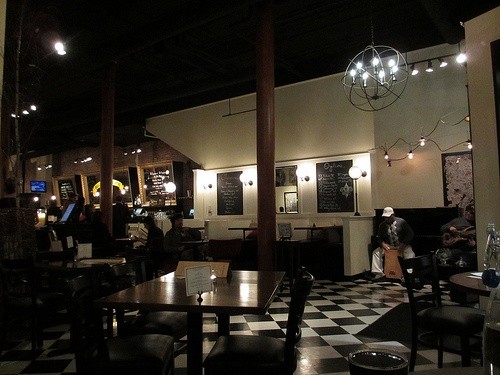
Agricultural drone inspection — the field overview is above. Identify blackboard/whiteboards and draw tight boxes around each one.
[316,160,354,212]
[217,171,244,215]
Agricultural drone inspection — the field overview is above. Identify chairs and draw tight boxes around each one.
[0,218,500,375]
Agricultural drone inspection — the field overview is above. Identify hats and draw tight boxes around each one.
[466,204,475,214]
[170,213,184,223]
[381,207,395,217]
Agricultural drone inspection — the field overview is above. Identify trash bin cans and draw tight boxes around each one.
[348,350,408,375]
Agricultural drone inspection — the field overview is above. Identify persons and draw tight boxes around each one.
[369,206,418,282]
[113,196,203,288]
[439,204,476,258]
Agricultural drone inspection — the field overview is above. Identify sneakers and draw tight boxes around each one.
[401,274,406,286]
[371,274,386,281]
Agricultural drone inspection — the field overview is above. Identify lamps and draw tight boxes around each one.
[239,173,253,186]
[419,137,426,146]
[408,150,414,159]
[200,180,213,189]
[466,140,472,149]
[342,0,410,112]
[348,166,366,180]
[425,60,434,73]
[456,52,467,63]
[384,152,389,159]
[437,58,448,68]
[296,168,310,182]
[410,64,419,75]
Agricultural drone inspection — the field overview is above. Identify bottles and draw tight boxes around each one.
[481,222,497,292]
[490,230,500,295]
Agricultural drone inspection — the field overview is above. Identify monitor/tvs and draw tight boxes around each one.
[59,200,81,226]
[30,180,46,192]
